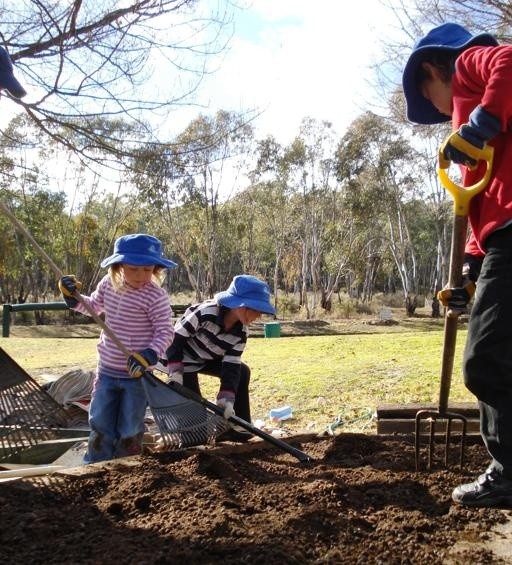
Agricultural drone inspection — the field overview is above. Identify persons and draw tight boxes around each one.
[155,275,275,442]
[0,46,27,99]
[58,234,178,465]
[403,23,512,505]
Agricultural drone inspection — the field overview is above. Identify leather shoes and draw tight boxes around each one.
[215,428,253,443]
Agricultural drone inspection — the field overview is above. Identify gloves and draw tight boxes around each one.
[444,106,501,172]
[126,348,157,377]
[167,361,184,386]
[58,275,82,308]
[217,387,235,419]
[436,263,476,317]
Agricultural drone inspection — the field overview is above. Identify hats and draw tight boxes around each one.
[215,275,275,315]
[101,234,179,269]
[403,23,499,124]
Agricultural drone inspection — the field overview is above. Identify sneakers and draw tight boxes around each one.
[452,468,512,506]
